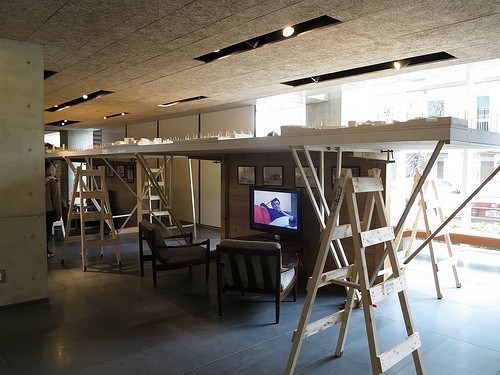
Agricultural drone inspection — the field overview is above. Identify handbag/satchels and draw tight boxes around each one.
[281,252,299,273]
[53,208,61,222]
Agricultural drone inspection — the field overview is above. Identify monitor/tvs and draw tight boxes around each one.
[249,185,303,244]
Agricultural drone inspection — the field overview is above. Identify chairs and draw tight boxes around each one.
[216,238,299,324]
[137,220,210,288]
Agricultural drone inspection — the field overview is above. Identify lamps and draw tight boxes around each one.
[394,60,410,71]
[247,40,258,49]
[311,77,320,82]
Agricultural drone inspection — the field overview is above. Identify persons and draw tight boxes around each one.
[260,198,296,227]
[45,158,61,258]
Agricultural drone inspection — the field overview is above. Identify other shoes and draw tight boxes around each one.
[48,251,54,258]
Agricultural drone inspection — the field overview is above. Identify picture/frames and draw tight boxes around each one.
[262,166,283,186]
[116,165,126,178]
[293,166,320,189]
[331,167,360,190]
[106,165,114,177]
[127,166,134,183]
[237,166,256,185]
